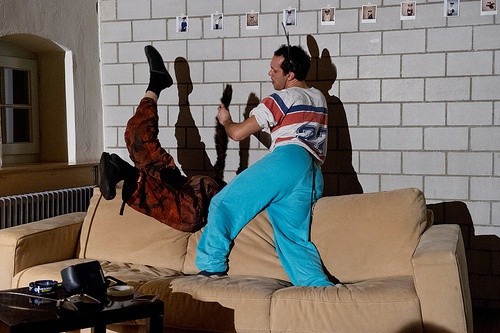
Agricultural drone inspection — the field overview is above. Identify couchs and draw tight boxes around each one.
[0,186,475,333]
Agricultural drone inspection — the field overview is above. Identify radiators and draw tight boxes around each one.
[0,185,97,229]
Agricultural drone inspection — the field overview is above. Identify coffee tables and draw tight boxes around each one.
[0,284,166,333]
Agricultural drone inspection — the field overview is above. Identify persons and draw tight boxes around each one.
[196,44,335,289]
[447,3,456,16]
[97,45,220,233]
[215,16,222,29]
[485,1,496,11]
[407,4,413,16]
[367,9,373,19]
[249,16,258,26]
[324,9,331,21]
[180,17,187,32]
[286,11,294,25]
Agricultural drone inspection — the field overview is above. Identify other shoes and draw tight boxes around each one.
[100,152,119,200]
[145,45,174,87]
